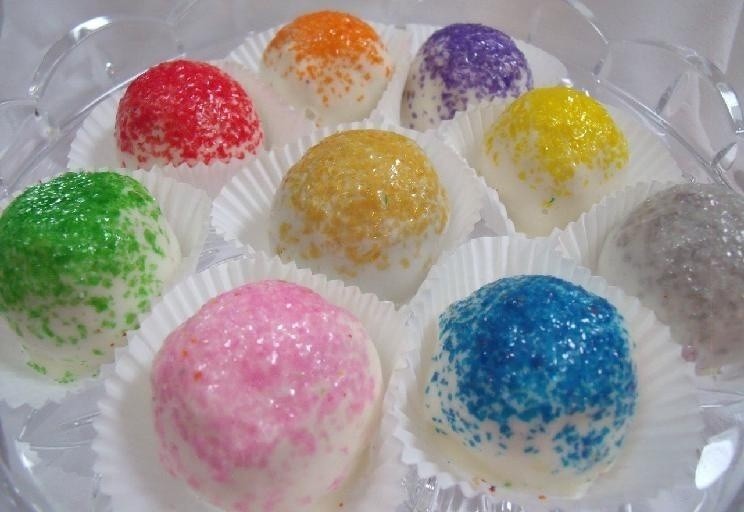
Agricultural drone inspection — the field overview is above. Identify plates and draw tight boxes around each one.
[0,0,744,511]
